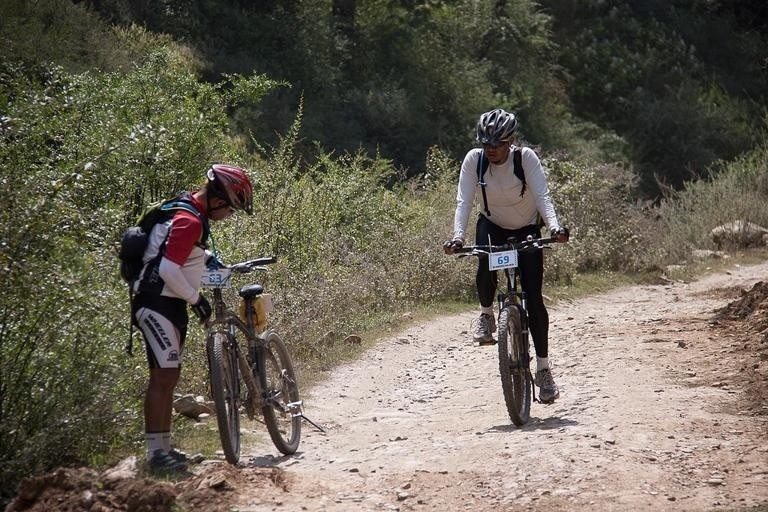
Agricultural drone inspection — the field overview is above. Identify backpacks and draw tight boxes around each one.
[120,201,209,283]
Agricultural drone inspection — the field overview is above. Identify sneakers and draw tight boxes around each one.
[474,313,497,341]
[148,447,205,473]
[534,369,558,403]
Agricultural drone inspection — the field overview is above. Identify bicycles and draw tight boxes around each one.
[201,253,326,465]
[447,226,569,427]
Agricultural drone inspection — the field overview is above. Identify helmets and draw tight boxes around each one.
[474,109,516,147]
[208,162,255,216]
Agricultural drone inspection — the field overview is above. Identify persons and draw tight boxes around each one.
[443,109,567,403]
[132,165,254,470]
